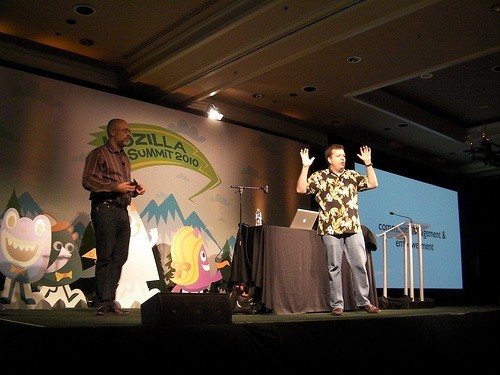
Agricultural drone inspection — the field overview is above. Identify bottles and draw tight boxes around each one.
[255,209,262,226]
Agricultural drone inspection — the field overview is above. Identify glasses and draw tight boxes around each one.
[116,129,131,134]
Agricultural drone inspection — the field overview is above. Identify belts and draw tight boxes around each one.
[97,201,124,207]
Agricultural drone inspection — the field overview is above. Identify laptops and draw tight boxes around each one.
[290,209,320,230]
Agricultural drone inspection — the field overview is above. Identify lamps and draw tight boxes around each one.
[207,103,224,120]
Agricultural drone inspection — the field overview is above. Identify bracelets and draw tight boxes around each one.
[365,163,372,167]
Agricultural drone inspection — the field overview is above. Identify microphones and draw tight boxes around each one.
[389,211,413,223]
[339,178,341,181]
[122,162,125,165]
[264,186,268,194]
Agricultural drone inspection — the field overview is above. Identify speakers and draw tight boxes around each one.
[141,293,232,326]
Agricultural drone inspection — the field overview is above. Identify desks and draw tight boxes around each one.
[231,225,380,316]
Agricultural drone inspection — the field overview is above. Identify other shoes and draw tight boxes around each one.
[95,306,106,316]
[109,307,130,314]
[358,303,381,312]
[332,308,342,316]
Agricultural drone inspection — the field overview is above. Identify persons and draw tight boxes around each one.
[81,118,145,316]
[295,144,382,315]
